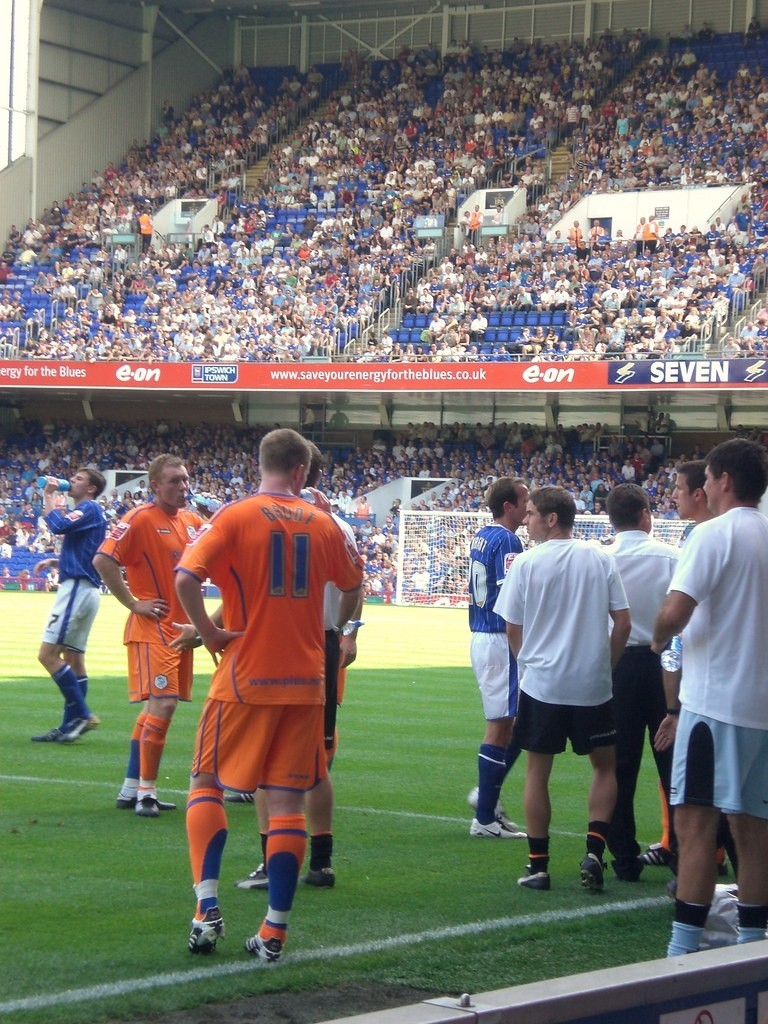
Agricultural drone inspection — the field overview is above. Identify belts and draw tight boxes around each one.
[619,646,654,654]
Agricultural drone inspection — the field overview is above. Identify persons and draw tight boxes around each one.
[464,476,531,840]
[603,481,688,883]
[92,452,209,819]
[633,17,768,357]
[165,420,279,521]
[168,428,367,961]
[650,439,768,958]
[0,415,168,593]
[491,488,631,896]
[311,27,644,363]
[0,64,324,361]
[28,469,108,742]
[459,410,768,600]
[298,404,458,603]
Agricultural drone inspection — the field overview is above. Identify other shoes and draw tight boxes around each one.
[57,713,101,742]
[31,730,64,743]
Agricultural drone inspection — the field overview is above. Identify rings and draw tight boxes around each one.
[153,608,160,614]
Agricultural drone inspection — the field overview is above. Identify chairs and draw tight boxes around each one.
[0,417,696,596]
[0,29,768,367]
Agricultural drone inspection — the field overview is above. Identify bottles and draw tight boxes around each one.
[301,489,316,504]
[661,634,682,672]
[341,620,361,635]
[37,477,70,492]
[185,494,220,513]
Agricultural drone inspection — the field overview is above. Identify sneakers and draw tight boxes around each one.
[467,788,518,832]
[517,866,550,889]
[233,863,269,890]
[136,795,161,817]
[116,793,177,810]
[637,842,677,866]
[243,934,282,962]
[579,853,607,895]
[470,817,528,839]
[299,868,336,888]
[185,906,224,954]
[223,790,253,802]
